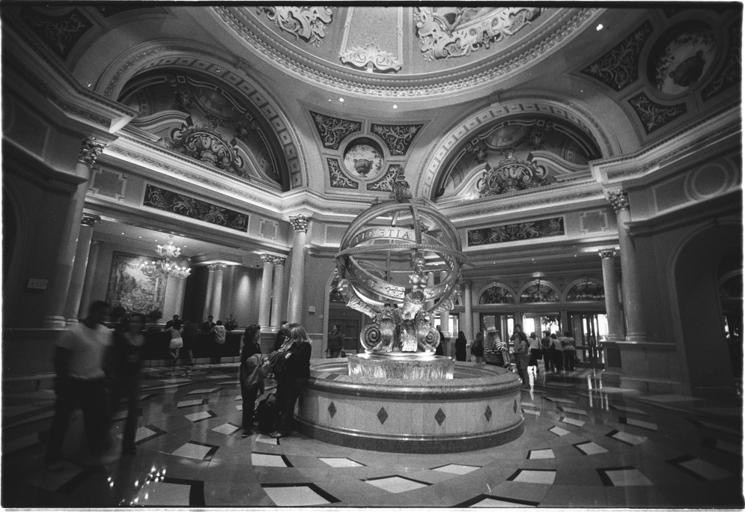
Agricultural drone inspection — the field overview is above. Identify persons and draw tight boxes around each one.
[202,319,226,364]
[328,324,345,358]
[164,316,184,355]
[46,302,144,460]
[239,321,312,437]
[436,324,577,390]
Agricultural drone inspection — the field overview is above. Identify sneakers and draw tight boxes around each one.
[240,430,253,438]
[269,430,283,438]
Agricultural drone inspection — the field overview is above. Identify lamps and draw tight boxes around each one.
[141,232,192,283]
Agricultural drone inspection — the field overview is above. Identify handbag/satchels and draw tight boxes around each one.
[256,393,279,431]
